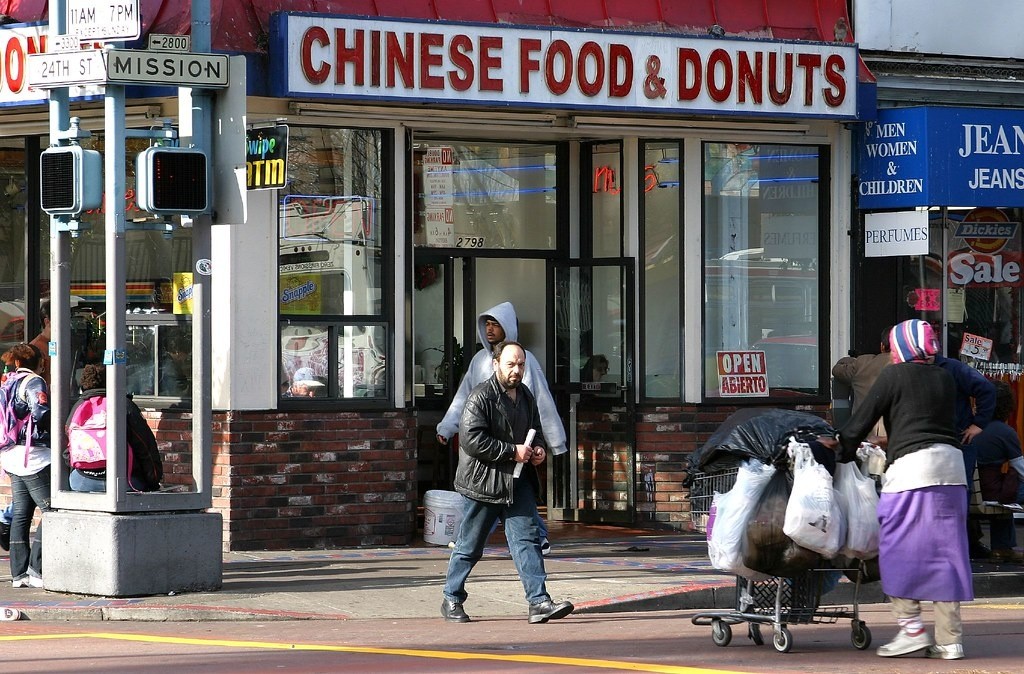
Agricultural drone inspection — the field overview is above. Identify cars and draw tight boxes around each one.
[709,334,818,398]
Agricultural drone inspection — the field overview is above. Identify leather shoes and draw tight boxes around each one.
[441,596,471,623]
[969,541,1024,564]
[528,601,574,624]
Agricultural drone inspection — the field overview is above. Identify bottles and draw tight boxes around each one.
[1,366,9,387]
[125,306,142,330]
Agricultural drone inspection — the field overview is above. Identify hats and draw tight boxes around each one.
[889,319,940,364]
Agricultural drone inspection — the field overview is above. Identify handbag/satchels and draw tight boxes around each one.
[684,408,836,582]
[783,445,882,559]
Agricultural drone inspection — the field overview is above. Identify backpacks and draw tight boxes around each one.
[0,372,33,452]
[68,396,106,469]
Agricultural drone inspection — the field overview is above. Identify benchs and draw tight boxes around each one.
[967,460,1024,521]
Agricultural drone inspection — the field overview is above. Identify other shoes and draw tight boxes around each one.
[12,575,43,588]
[540,537,551,556]
[448,541,457,548]
[0,523,11,551]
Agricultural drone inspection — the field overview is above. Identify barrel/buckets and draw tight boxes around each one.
[424,490,465,545]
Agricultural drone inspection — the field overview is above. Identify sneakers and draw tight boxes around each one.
[876,627,933,657]
[924,644,965,660]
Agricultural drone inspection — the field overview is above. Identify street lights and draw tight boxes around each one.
[153,276,172,308]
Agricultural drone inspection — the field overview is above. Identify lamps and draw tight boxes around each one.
[289,101,556,127]
[568,116,811,137]
[0,106,161,129]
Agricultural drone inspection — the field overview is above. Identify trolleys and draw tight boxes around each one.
[688,441,878,654]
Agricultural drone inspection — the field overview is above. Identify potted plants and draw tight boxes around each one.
[420,337,484,397]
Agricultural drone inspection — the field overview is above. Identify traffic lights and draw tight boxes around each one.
[40,145,102,216]
[135,146,213,216]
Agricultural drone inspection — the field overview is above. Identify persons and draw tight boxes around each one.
[583,353,609,382]
[436,301,575,625]
[813,318,1024,661]
[0,296,192,587]
[281,367,326,397]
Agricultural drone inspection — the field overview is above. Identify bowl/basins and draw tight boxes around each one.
[427,384,443,395]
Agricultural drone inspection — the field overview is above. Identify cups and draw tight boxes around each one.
[414,384,425,397]
[415,365,425,383]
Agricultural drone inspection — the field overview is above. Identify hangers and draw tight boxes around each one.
[973,360,1024,382]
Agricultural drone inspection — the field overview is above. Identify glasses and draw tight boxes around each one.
[596,367,611,373]
